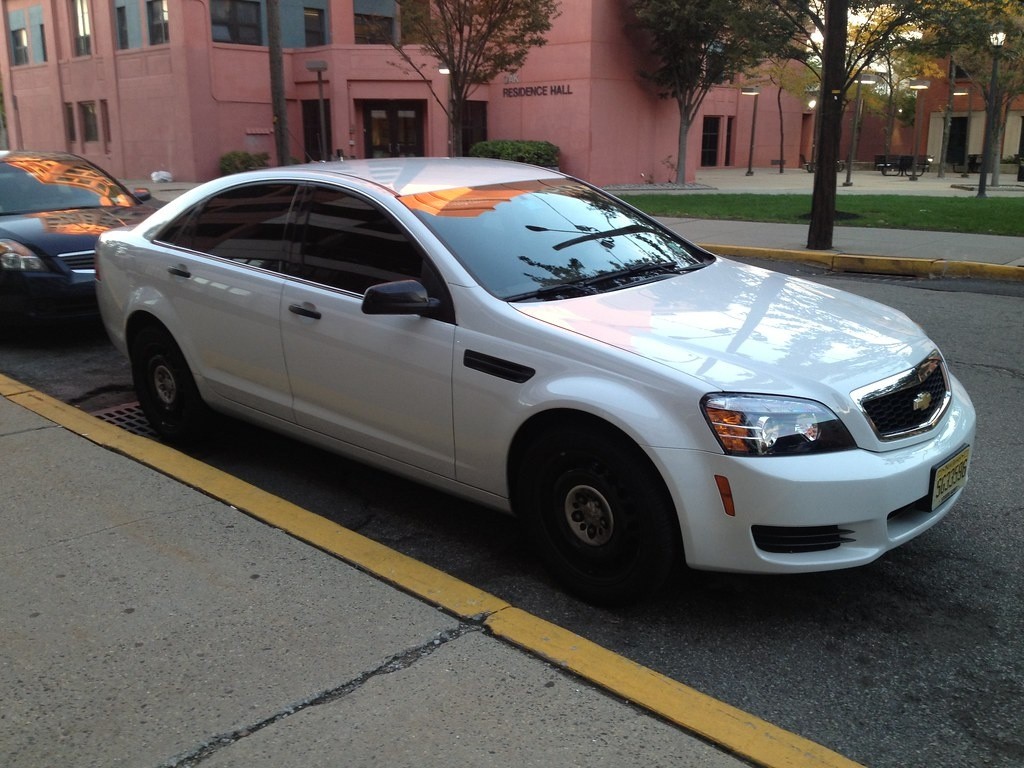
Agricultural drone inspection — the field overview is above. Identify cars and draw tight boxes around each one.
[92,155,978,611]
[0,149,167,331]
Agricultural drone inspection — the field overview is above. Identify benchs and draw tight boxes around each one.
[967,153,984,172]
[873,152,933,177]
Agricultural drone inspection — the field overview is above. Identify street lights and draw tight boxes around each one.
[975,30,1007,200]
[741,86,759,177]
[303,60,328,162]
[907,79,930,180]
[841,73,879,187]
[437,62,454,159]
[953,86,971,179]
